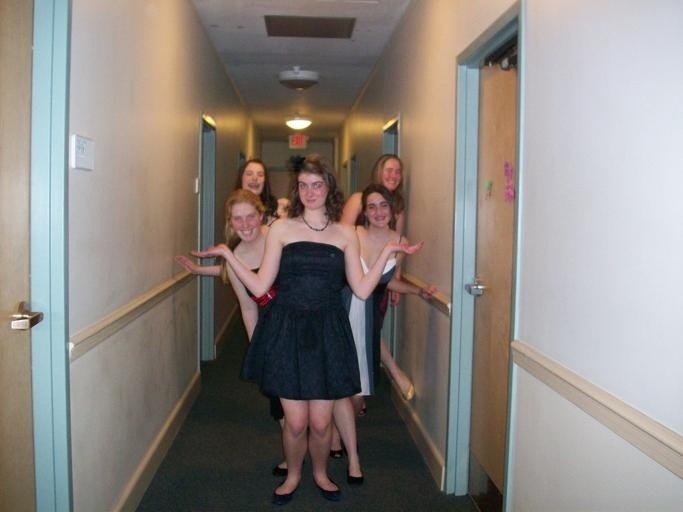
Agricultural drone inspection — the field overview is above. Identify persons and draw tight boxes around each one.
[174,153,436,507]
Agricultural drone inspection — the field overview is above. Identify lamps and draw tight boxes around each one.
[285,117,313,130]
[276,70,319,92]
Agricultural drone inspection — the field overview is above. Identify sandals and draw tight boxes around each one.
[272,448,365,504]
[358,407,367,417]
